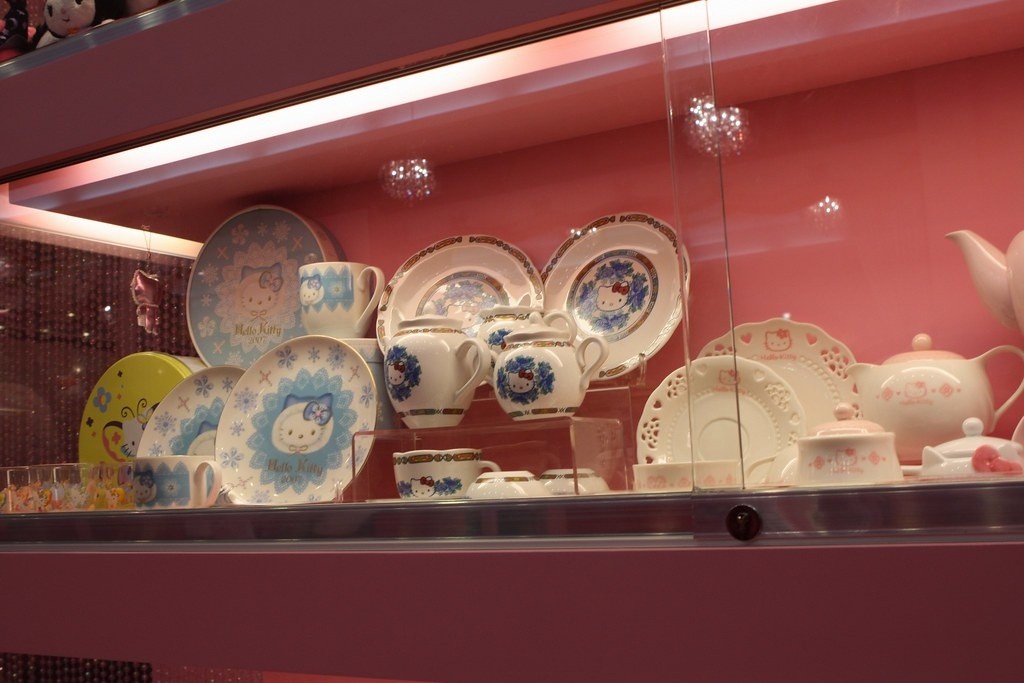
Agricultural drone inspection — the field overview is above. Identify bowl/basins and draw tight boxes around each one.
[535,469,609,495]
[468,468,538,498]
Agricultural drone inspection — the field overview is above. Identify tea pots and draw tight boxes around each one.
[918,417,1024,478]
[383,314,492,428]
[477,294,576,387]
[492,312,610,422]
[844,333,1024,474]
[946,230,1024,336]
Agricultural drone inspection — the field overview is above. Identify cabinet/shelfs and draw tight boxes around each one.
[0,0,1024,683]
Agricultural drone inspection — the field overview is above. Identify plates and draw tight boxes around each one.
[541,211,689,380]
[697,316,863,433]
[376,233,547,389]
[214,335,378,505]
[185,204,350,368]
[636,355,807,490]
[135,365,247,455]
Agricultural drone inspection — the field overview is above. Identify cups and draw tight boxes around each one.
[0,463,102,514]
[130,454,223,509]
[631,459,739,491]
[393,448,501,501]
[746,438,807,492]
[297,261,385,339]
[780,403,903,485]
